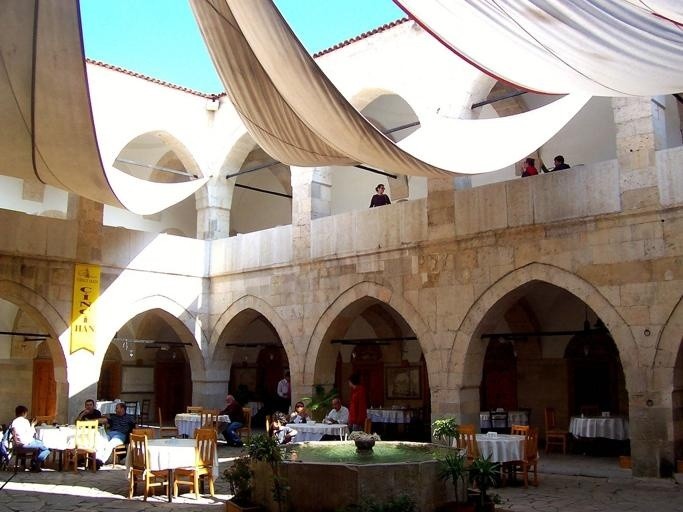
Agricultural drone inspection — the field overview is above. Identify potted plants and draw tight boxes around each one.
[431,416,474,512]
[468,451,503,512]
[223,459,259,512]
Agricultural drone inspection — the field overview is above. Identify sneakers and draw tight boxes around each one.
[87,457,92,469]
[232,442,242,446]
[90,459,100,470]
[224,443,231,446]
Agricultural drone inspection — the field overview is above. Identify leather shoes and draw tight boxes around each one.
[31,460,42,471]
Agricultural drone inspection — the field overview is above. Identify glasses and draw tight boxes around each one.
[379,188,386,190]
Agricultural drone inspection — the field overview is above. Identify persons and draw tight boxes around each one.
[369,184,391,208]
[219,395,246,447]
[290,402,310,424]
[520,158,539,178]
[271,412,297,445]
[277,370,291,414]
[75,399,102,426]
[541,156,571,173]
[323,398,349,424]
[88,403,139,471]
[347,373,367,431]
[9,406,51,472]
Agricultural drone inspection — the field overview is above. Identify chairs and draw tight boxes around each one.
[14,428,39,470]
[132,428,157,496]
[501,427,540,491]
[236,407,252,439]
[105,416,128,468]
[363,416,372,433]
[63,420,98,473]
[455,425,481,470]
[543,405,570,457]
[159,426,180,494]
[186,407,203,414]
[35,415,57,468]
[265,415,272,438]
[174,428,215,500]
[510,424,530,436]
[158,407,178,439]
[137,398,151,427]
[128,432,169,502]
[124,400,138,423]
[200,410,218,438]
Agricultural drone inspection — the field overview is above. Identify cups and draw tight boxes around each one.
[607,411,610,417]
[602,411,607,417]
[41,422,46,429]
